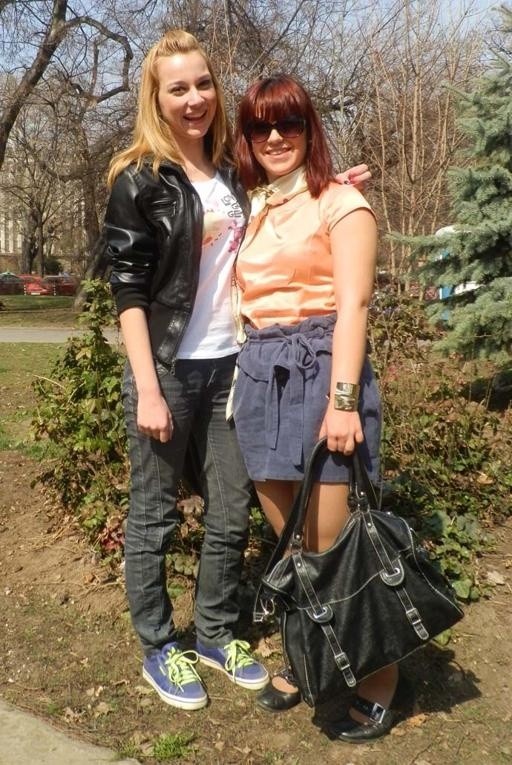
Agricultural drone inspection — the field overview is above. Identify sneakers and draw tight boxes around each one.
[193,631,271,690]
[142,639,211,710]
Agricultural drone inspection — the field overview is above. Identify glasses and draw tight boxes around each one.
[244,112,309,144]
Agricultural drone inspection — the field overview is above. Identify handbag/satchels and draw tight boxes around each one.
[248,428,464,708]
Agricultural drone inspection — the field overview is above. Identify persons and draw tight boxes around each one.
[219,67,408,744]
[98,27,374,711]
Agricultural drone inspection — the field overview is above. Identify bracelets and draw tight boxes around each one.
[334,380,363,412]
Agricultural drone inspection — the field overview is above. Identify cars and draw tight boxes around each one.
[0,272,80,298]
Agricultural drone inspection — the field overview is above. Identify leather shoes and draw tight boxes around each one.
[256,666,306,714]
[325,668,417,742]
